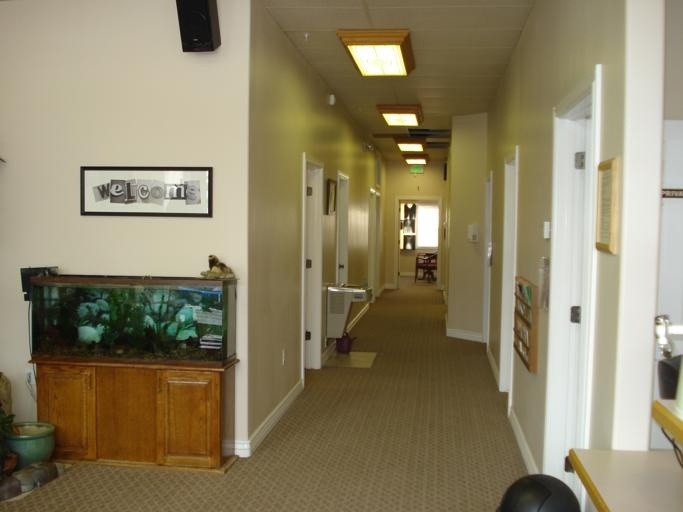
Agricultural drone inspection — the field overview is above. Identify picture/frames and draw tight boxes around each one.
[79,166,212,218]
[325,178,337,215]
[512,275,538,376]
[594,157,620,256]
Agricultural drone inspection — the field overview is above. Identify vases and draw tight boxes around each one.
[5,422,54,468]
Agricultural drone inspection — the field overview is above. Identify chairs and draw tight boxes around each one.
[417,250,438,281]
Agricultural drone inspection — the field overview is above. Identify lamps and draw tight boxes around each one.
[336,27,415,78]
[391,136,426,152]
[402,154,428,166]
[375,103,423,128]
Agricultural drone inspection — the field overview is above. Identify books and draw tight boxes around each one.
[514,280,532,366]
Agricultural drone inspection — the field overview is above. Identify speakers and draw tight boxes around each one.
[176,0,221,52]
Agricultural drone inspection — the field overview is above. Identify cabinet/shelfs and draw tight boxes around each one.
[568,398,682,512]
[30,354,241,474]
